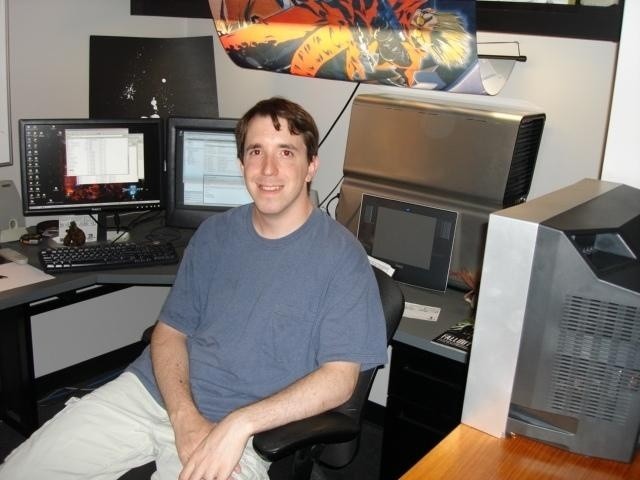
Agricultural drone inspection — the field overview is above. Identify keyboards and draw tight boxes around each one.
[38,243,179,275]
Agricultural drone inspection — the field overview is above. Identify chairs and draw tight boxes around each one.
[141,263,406,480]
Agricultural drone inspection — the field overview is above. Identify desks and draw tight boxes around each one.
[0,224,475,438]
[394,422,640,480]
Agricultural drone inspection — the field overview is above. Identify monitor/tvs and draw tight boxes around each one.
[18,117,167,249]
[460,178,640,466]
[355,192,458,293]
[164,117,255,229]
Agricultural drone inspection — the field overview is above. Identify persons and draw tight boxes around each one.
[0,96,389,480]
